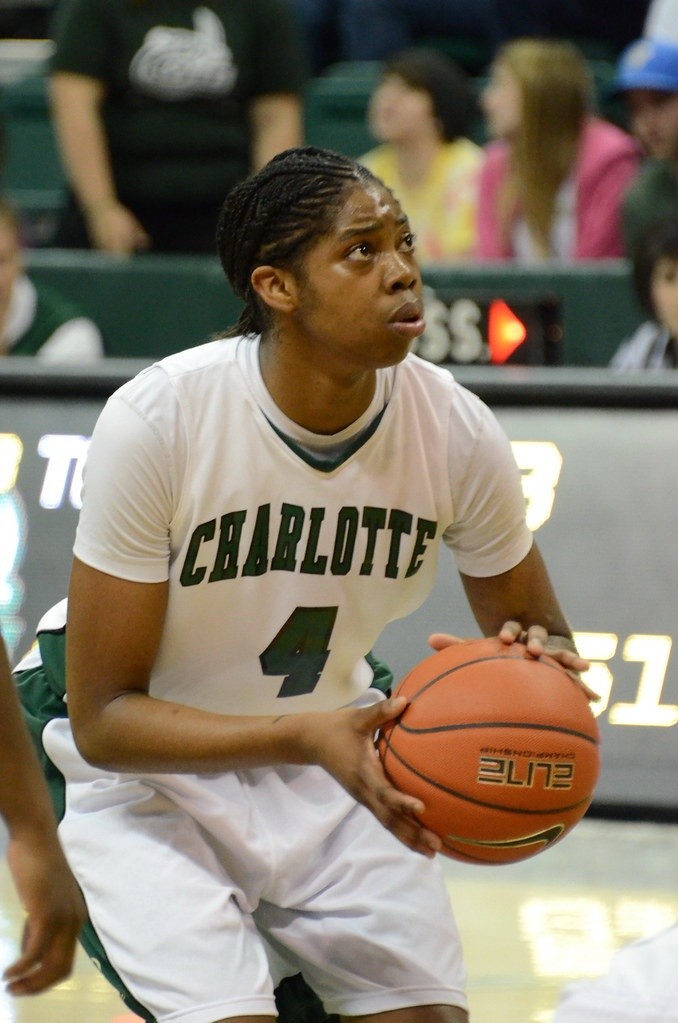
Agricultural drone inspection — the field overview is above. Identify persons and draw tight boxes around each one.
[0,0,677,1022]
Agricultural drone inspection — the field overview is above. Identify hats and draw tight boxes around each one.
[599,36,678,124]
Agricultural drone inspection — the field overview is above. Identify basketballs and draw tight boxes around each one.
[375,633,602,869]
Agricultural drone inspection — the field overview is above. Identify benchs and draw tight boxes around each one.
[0,63,678,366]
